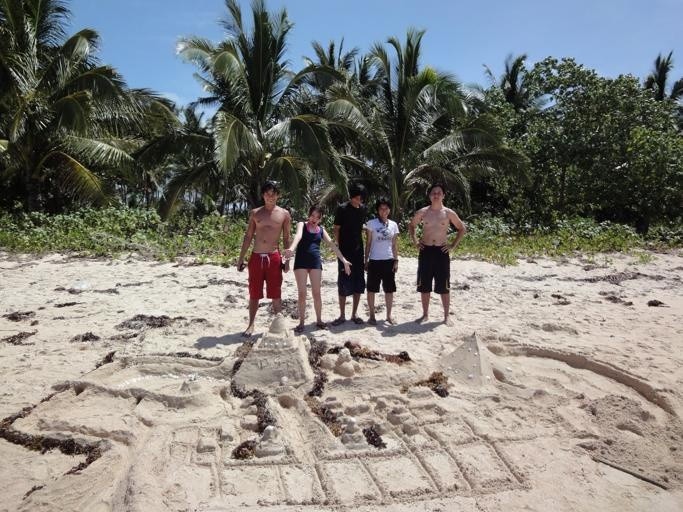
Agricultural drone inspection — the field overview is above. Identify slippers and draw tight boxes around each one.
[295,318,363,332]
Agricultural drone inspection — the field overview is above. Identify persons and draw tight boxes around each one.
[409,183,466,328]
[365,195,400,326]
[236,180,292,338]
[331,182,369,325]
[285,204,353,334]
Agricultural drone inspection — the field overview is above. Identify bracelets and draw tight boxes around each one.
[283,258,292,261]
[394,259,399,261]
